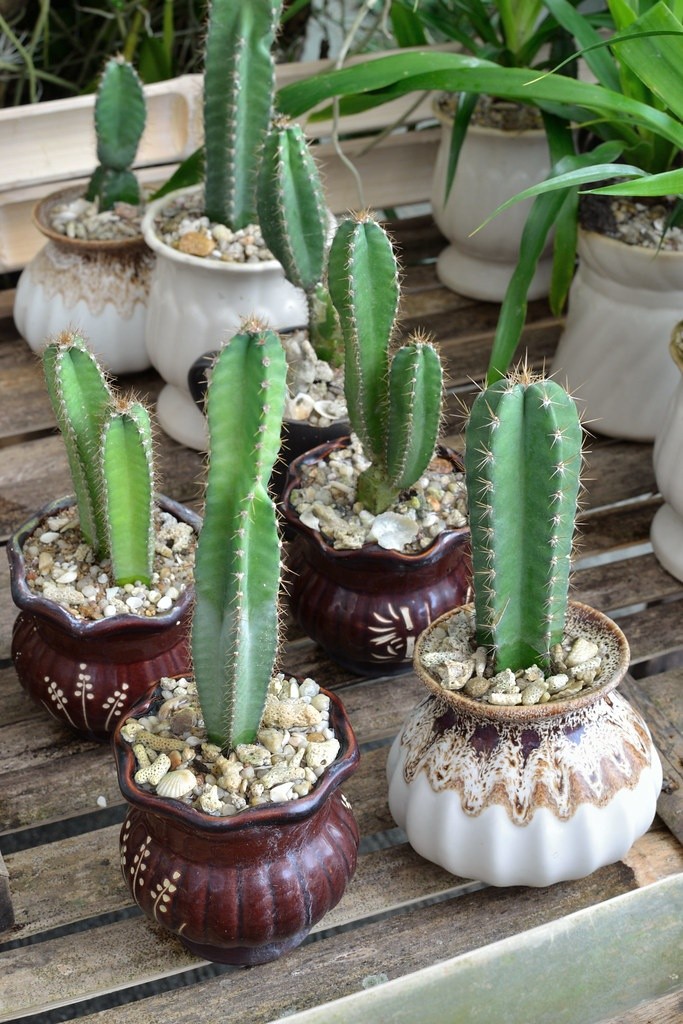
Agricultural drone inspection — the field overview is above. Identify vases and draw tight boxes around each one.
[649,322,683,583]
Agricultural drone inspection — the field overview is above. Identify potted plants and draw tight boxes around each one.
[114,313,359,967]
[7,332,203,732]
[140,0,339,454]
[155,2,683,452]
[12,56,155,375]
[388,359,661,888]
[279,208,476,680]
[189,117,349,498]
